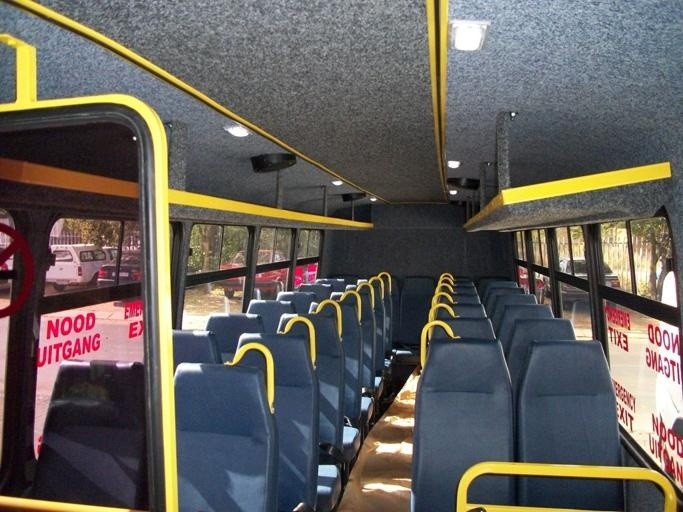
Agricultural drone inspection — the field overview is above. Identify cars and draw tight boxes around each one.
[518,263,546,305]
[218,247,291,301]
[97,249,141,292]
[541,257,622,312]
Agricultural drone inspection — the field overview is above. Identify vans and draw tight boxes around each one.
[44,243,116,291]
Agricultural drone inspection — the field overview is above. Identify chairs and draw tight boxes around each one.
[400,272,620,512]
[33,272,400,512]
